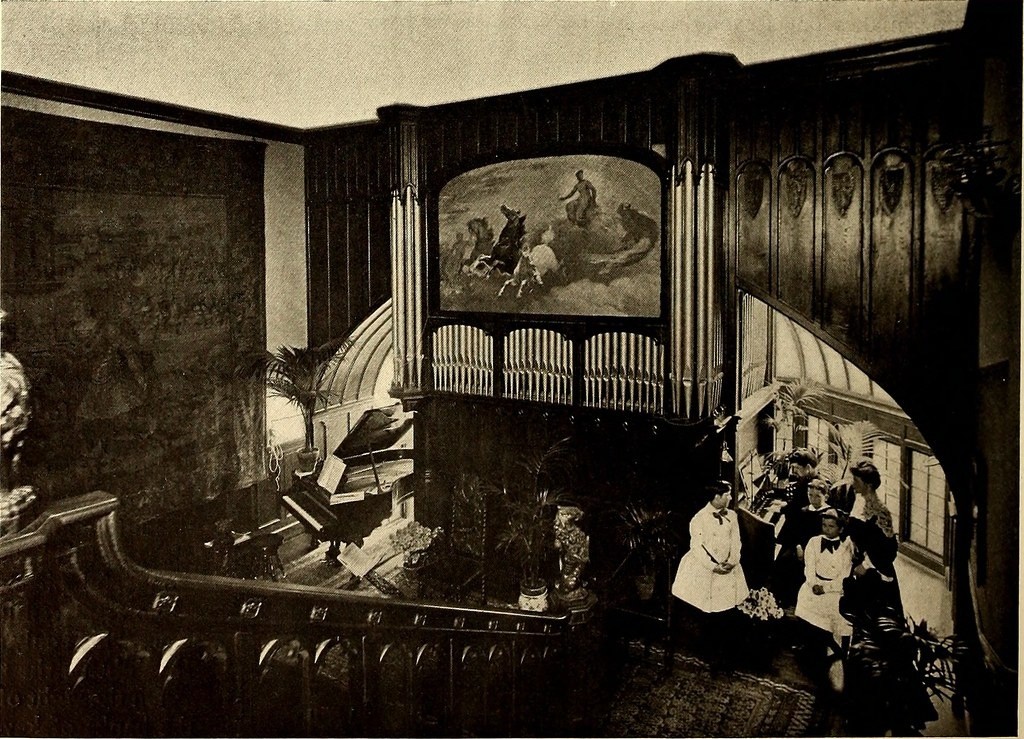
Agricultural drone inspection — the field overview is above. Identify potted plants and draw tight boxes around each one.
[616,498,676,602]
[481,435,586,614]
[761,375,830,485]
[234,336,339,473]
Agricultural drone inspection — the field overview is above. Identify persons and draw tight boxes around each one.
[782,451,941,737]
[671,479,750,669]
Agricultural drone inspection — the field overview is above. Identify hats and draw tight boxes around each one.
[849,461,881,490]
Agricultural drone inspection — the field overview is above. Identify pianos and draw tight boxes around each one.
[283,399,418,562]
[739,461,797,588]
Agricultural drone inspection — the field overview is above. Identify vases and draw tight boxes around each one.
[400,560,425,587]
[748,622,773,652]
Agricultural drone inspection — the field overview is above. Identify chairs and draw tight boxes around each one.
[404,483,488,607]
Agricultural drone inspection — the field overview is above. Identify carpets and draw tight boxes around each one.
[277,553,816,738]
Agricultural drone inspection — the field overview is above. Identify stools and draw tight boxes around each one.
[248,530,285,584]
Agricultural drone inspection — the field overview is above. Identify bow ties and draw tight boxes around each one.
[820,537,840,555]
[713,509,730,526]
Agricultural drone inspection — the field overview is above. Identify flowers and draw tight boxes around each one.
[737,587,785,622]
[390,517,438,567]
